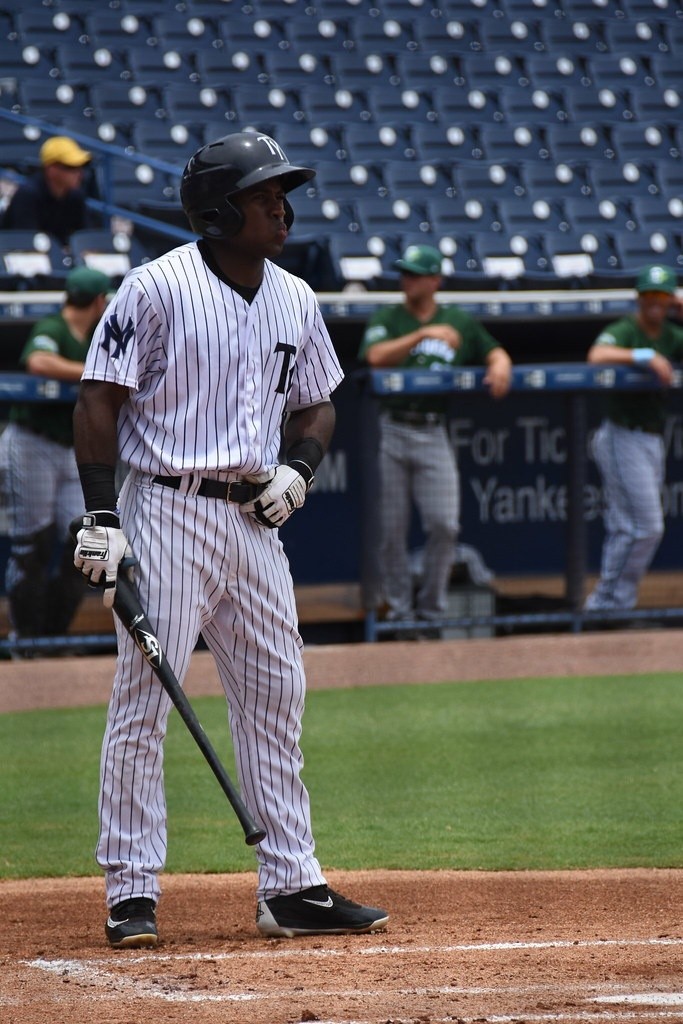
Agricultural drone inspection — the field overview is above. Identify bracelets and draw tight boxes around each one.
[633,347,655,368]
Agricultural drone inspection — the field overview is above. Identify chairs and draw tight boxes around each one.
[0,0,683,296]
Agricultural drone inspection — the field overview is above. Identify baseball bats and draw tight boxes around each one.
[99,574,266,846]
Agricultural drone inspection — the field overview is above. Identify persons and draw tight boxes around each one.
[0,267,113,659]
[584,265,683,608]
[361,245,511,640]
[0,136,90,239]
[74,133,388,949]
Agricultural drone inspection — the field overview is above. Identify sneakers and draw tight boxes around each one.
[105,897,158,945]
[254,885,389,938]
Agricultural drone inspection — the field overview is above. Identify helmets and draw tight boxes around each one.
[180,132,316,240]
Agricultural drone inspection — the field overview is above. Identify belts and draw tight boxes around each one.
[631,419,664,434]
[389,411,445,426]
[152,475,268,503]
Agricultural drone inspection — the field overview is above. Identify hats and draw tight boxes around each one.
[392,244,442,274]
[43,137,92,167]
[637,263,676,294]
[67,267,110,297]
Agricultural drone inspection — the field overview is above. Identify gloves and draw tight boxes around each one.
[239,460,316,528]
[72,510,136,609]
